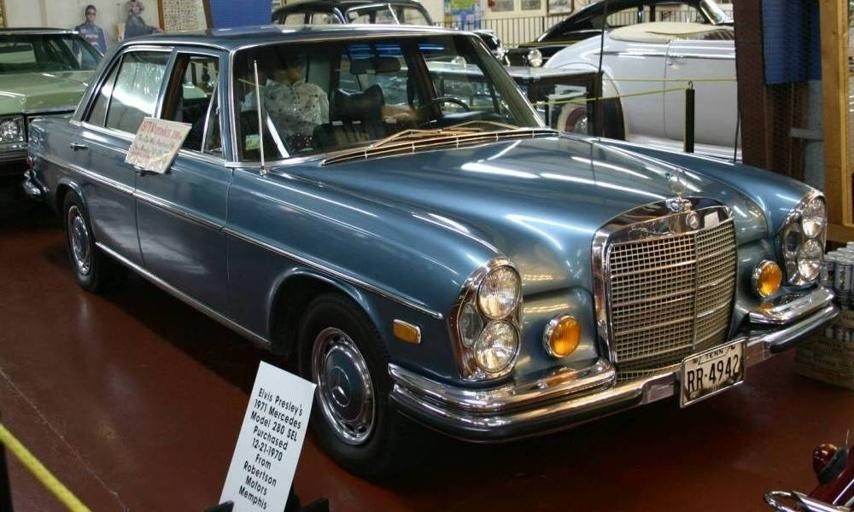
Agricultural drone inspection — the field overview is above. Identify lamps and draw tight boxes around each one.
[188,81,278,164]
[312,84,401,151]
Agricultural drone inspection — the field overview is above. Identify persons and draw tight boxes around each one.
[72,5,106,69]
[240,44,330,158]
[122,0,163,40]
